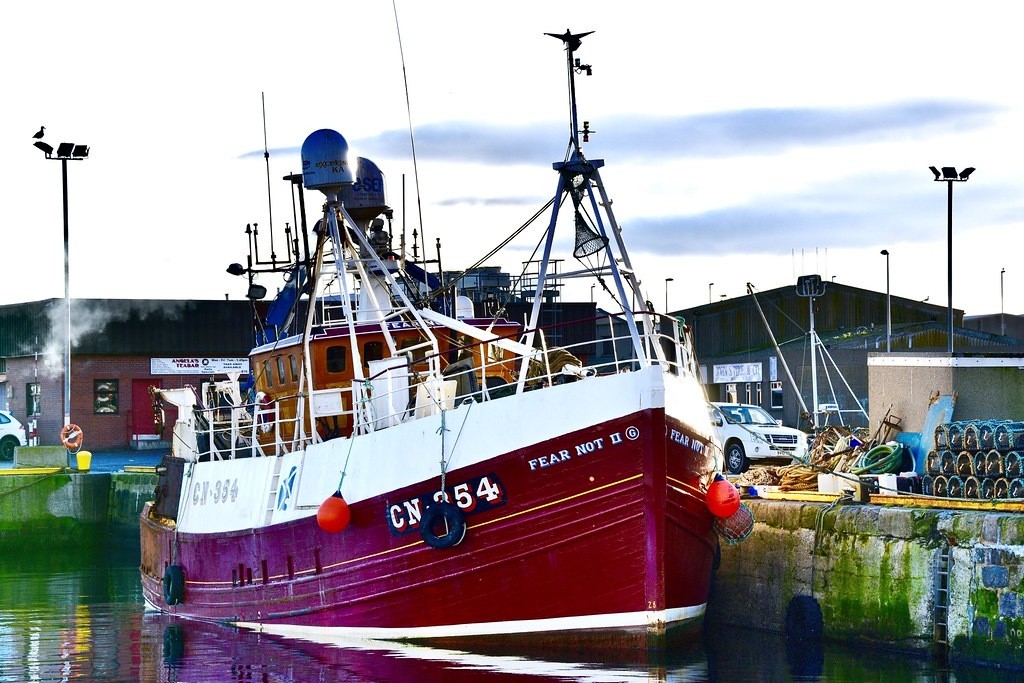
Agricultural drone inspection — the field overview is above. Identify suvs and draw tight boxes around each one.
[0,409,28,461]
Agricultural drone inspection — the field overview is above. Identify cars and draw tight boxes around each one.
[708,401,809,474]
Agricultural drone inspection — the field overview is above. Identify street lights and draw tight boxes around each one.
[929,164,977,352]
[1000,271,1006,337]
[32,141,92,428]
[880,249,893,352]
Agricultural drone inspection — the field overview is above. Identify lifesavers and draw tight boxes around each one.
[163,626,183,658]
[254,390,275,433]
[61,424,83,449]
[419,503,466,551]
[784,595,824,640]
[162,565,185,605]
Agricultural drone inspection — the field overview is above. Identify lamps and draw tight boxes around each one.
[831,276,836,283]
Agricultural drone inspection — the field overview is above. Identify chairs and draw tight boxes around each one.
[732,414,742,423]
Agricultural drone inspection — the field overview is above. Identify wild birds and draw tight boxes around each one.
[32,125,46,141]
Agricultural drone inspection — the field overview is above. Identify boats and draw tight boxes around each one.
[138,0,727,645]
